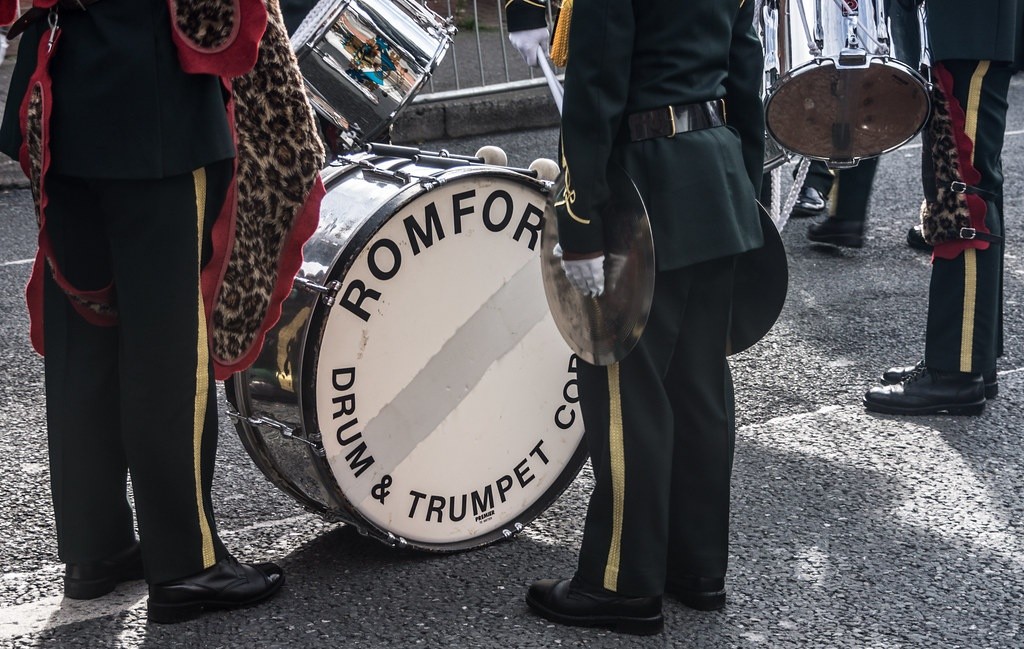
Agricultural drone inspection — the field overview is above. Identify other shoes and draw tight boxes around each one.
[792,187,830,217]
[807,216,862,256]
[908,225,929,248]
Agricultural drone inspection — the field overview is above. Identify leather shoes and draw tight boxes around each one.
[864,364,986,417]
[664,580,727,611]
[877,366,998,398]
[526,575,665,634]
[147,555,286,623]
[63,550,143,599]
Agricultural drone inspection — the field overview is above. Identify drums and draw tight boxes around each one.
[754,0,937,171]
[761,128,798,174]
[221,136,598,556]
[288,0,460,147]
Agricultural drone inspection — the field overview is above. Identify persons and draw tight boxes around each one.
[0,0,326,626]
[790,153,880,247]
[504,0,765,631]
[863,0,1018,415]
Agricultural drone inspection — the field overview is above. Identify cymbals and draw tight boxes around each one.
[537,162,660,369]
[725,197,790,359]
